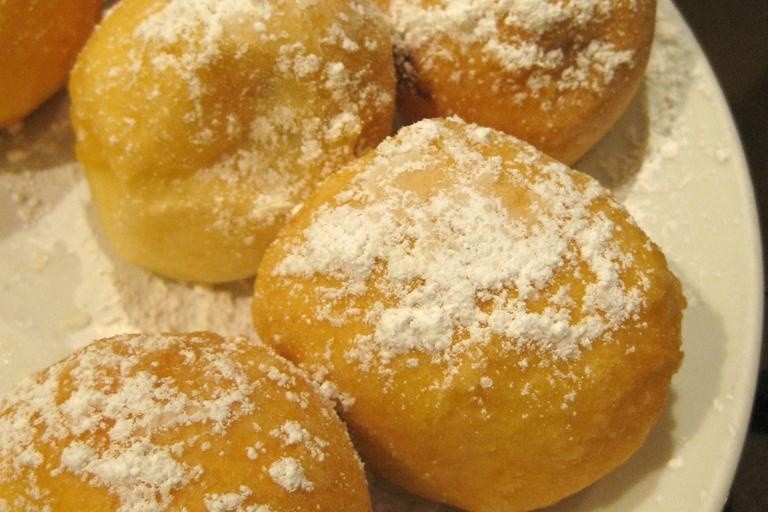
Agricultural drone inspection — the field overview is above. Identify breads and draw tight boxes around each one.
[2,0,101,132]
[379,1,658,169]
[251,118,689,511]
[0,331,373,512]
[68,0,400,287]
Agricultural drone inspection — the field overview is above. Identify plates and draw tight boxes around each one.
[0,0,767,512]
